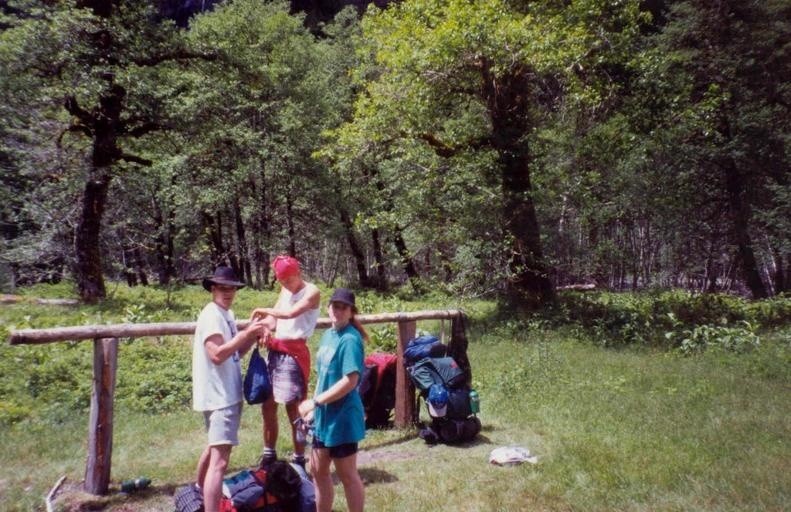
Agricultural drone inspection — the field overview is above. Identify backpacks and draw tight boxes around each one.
[349,351,400,431]
[403,332,486,448]
[171,457,319,512]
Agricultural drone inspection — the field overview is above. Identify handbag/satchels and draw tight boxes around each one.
[242,335,272,405]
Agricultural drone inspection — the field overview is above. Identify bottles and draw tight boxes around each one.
[295,420,306,444]
[121,479,151,492]
[469,388,480,413]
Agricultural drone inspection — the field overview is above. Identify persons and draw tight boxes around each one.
[190,265,269,512]
[250,254,322,472]
[297,290,373,512]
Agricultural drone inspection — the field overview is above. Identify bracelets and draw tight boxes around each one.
[312,396,321,408]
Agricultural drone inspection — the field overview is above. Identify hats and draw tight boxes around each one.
[203,266,247,291]
[272,255,300,280]
[327,286,361,312]
